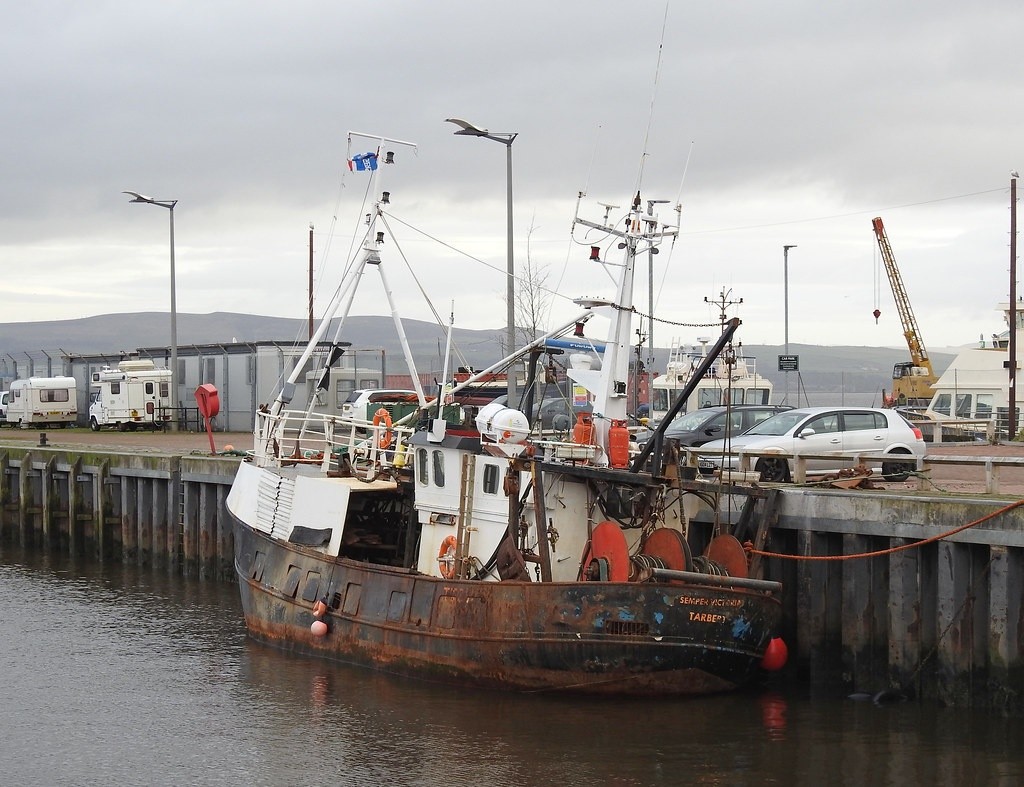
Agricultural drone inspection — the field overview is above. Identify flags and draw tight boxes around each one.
[346,152,378,173]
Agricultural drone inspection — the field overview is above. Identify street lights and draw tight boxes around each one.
[121,190,180,434]
[447,115,517,412]
[782,243,797,408]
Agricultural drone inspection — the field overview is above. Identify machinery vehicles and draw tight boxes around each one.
[871,216,942,420]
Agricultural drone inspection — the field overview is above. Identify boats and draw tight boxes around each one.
[924,294,1023,441]
[223,119,785,700]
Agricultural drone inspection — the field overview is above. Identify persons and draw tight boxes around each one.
[636,402,648,426]
[538,438,547,456]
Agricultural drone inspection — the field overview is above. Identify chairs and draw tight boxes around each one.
[812,419,825,434]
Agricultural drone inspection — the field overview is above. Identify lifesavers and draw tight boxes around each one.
[439,535,459,579]
[371,408,393,449]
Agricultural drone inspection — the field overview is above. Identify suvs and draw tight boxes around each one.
[341,387,416,434]
[628,404,798,447]
[697,407,927,483]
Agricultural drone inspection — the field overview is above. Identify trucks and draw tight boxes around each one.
[88,359,173,433]
[7,376,78,428]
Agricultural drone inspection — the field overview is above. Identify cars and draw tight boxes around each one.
[523,397,592,432]
[0,390,9,418]
[484,394,551,408]
